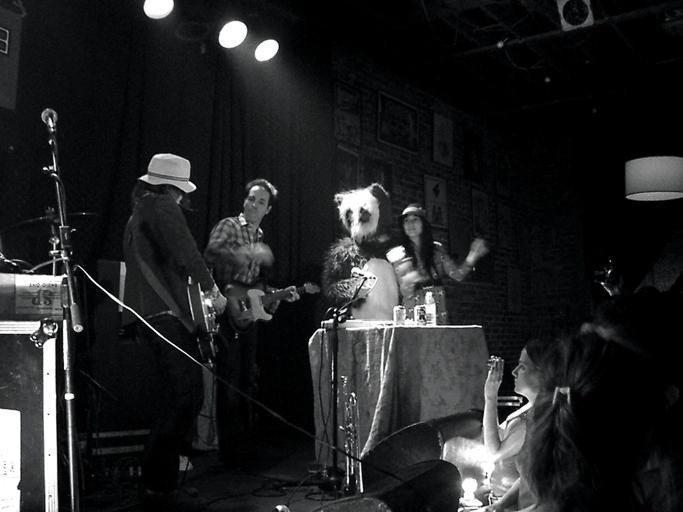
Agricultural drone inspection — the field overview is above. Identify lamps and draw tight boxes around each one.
[622,153,682,204]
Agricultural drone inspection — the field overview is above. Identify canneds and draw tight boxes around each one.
[414,304,427,326]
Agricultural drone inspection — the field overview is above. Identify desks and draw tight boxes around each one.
[305,316,493,494]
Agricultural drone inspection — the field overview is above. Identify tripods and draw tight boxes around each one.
[272,279,376,501]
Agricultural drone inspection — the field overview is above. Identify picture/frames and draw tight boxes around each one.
[329,76,528,319]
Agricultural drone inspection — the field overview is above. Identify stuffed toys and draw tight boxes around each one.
[321,183,424,321]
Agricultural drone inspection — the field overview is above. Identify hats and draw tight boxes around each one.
[397,203,428,225]
[137,153,197,194]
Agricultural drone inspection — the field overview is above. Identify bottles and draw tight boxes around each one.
[423,291,437,325]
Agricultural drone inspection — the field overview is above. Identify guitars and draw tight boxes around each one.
[226,282,320,328]
[186,269,219,362]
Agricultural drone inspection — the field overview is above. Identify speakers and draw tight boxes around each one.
[96,258,186,452]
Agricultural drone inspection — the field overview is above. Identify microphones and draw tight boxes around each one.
[350,266,376,280]
[42,107,58,130]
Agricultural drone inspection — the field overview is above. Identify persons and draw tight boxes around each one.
[123,153,229,496]
[399,202,489,324]
[203,178,301,474]
[442,278,683,512]
